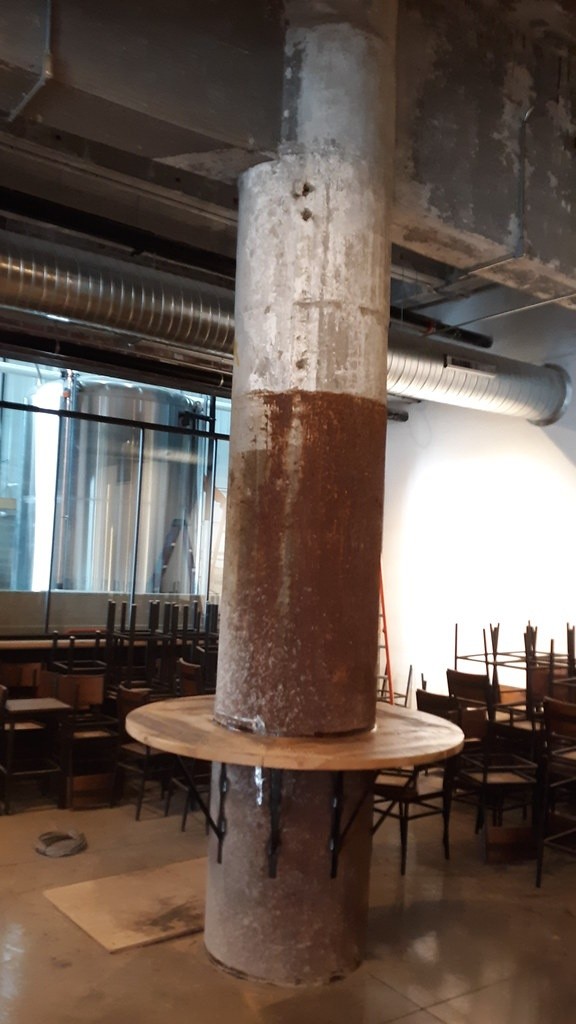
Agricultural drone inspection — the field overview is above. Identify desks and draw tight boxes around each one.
[125,694,463,876]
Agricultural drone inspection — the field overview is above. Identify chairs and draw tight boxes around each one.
[0,598,576,889]
